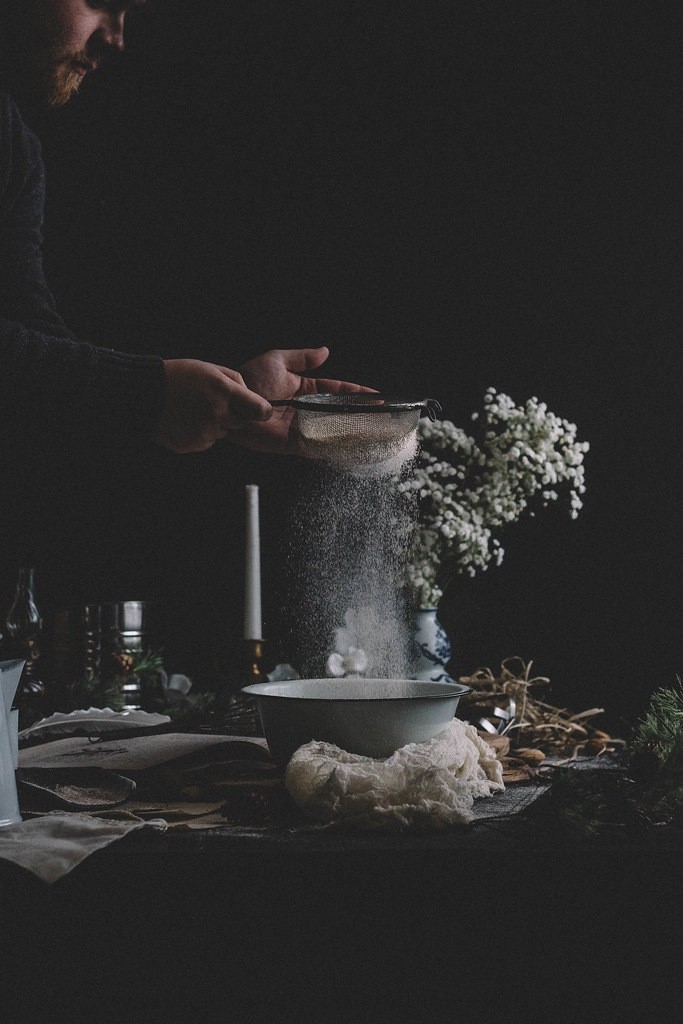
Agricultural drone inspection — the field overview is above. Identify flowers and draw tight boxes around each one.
[396,387,588,607]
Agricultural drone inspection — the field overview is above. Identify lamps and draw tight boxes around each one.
[6,569,46,732]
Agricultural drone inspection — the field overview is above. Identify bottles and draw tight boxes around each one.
[5,567,40,638]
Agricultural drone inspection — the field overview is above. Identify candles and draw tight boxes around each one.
[246,484,262,640]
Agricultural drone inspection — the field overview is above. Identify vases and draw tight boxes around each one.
[408,608,459,685]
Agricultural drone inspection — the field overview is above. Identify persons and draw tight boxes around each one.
[0,0,384,455]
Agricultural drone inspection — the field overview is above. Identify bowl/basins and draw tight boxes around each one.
[241,677,475,770]
